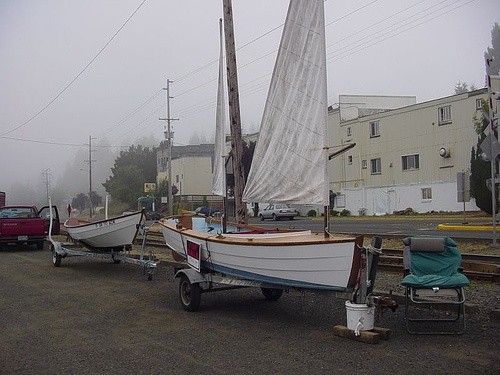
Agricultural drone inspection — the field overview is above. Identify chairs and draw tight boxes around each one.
[403,236,470,336]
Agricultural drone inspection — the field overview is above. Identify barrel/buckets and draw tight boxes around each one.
[345,299,375,330]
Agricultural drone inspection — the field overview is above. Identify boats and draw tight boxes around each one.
[63,205,145,255]
[155,212,365,295]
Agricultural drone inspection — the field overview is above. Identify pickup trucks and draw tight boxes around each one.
[0,205,62,252]
[257,204,297,222]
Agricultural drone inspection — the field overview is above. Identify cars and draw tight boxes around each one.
[195,206,219,218]
[45,211,54,218]
